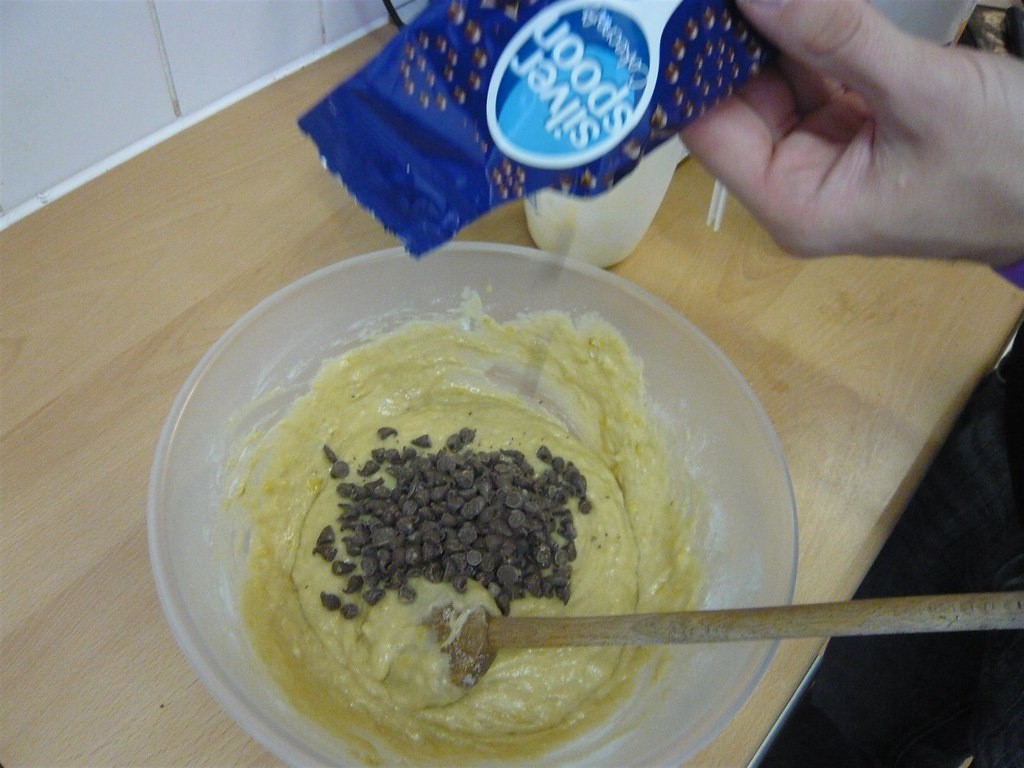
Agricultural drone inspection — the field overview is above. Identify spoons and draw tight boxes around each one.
[415,591,1024,709]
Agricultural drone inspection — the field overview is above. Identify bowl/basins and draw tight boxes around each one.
[145,241,801,768]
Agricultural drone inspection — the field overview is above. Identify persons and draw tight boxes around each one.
[677,0,1024,296]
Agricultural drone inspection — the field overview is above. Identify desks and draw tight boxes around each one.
[0,1,1023,767]
